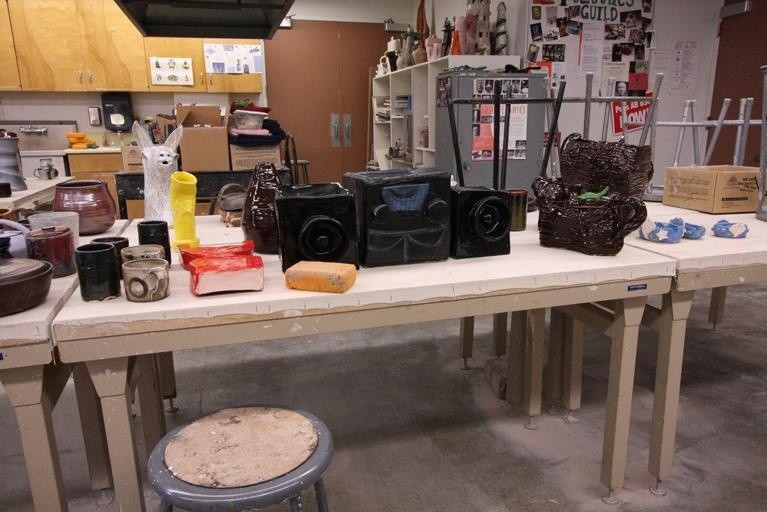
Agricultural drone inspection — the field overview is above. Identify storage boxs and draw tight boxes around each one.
[665,161,763,214]
[121,103,281,173]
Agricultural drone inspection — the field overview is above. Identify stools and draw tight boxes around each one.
[445,72,767,222]
[147,403,333,512]
[283,160,310,186]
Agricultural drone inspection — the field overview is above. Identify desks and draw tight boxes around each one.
[0,217,124,512]
[528,202,767,496]
[116,165,292,219]
[53,215,675,505]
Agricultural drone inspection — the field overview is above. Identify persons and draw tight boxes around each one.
[617,83,626,96]
[624,14,637,28]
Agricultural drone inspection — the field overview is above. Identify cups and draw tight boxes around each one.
[34,158,56,180]
[74,220,172,303]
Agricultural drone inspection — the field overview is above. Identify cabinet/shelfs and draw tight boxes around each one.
[68,155,146,219]
[0,1,263,95]
[372,55,521,172]
